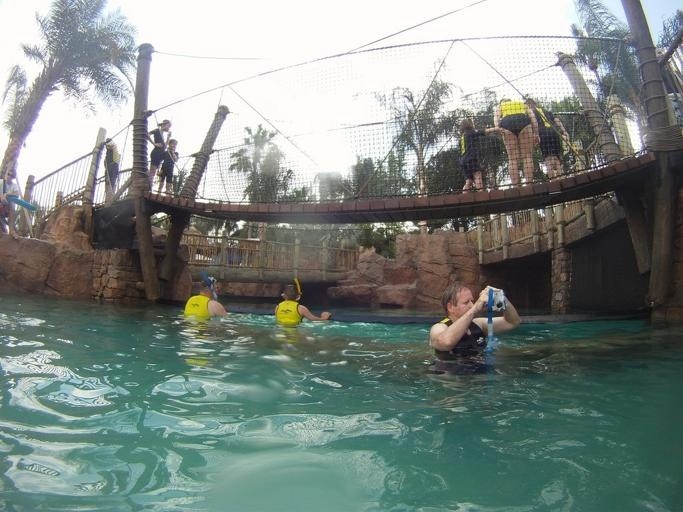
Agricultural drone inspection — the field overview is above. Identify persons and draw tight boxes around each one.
[158,139,179,195]
[184,276,228,320]
[494,97,540,188]
[525,99,570,179]
[430,283,521,373]
[101,137,122,204]
[459,118,500,193]
[147,119,171,192]
[274,278,332,324]
[0,169,20,233]
